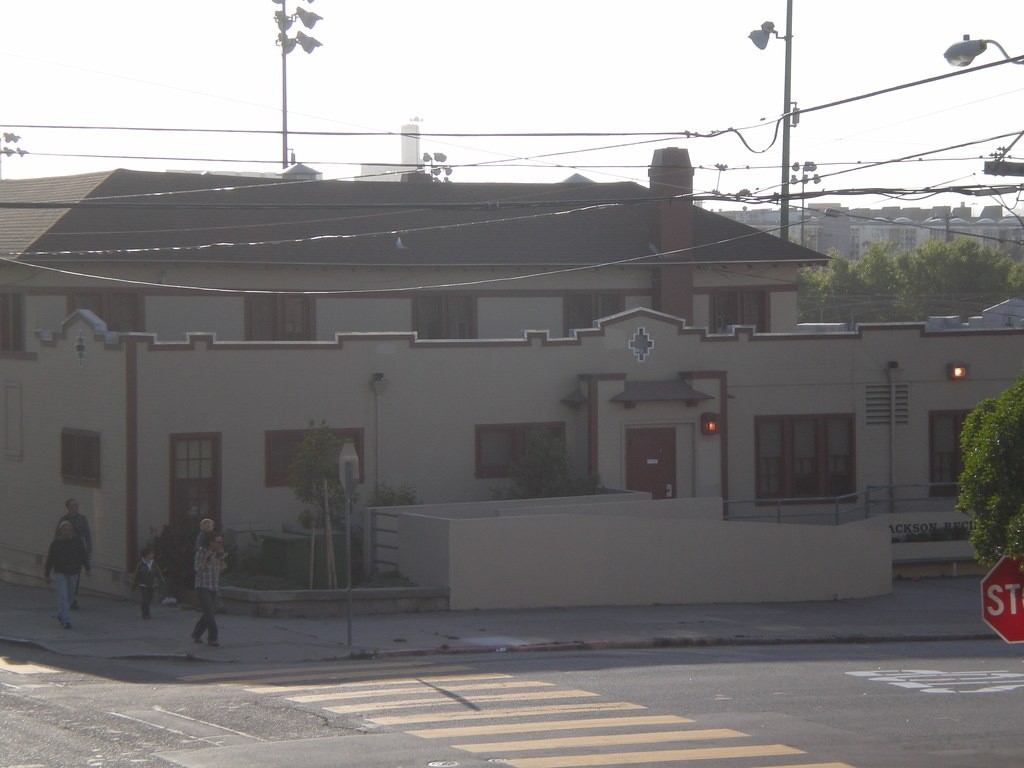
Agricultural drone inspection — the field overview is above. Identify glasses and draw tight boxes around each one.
[216,541,223,544]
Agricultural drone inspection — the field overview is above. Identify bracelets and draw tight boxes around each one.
[86,567,90,570]
[207,550,214,554]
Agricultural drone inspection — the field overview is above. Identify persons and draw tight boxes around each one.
[190,532,228,647]
[54,498,92,610]
[129,546,164,619]
[193,518,228,560]
[44,521,92,627]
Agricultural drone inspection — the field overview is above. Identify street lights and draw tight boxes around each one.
[272,0,323,169]
[789,161,822,248]
[748,0,794,243]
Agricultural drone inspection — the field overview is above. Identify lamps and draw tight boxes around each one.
[702,412,719,436]
[950,362,967,379]
[75,330,85,367]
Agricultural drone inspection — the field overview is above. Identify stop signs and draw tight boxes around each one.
[981,554,1024,644]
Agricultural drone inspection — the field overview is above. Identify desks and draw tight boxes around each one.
[259,528,347,581]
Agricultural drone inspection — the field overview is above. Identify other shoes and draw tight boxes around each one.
[190,632,203,643]
[62,622,71,629]
[141,614,152,620]
[207,639,220,647]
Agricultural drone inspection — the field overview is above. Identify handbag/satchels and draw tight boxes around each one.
[162,594,178,609]
